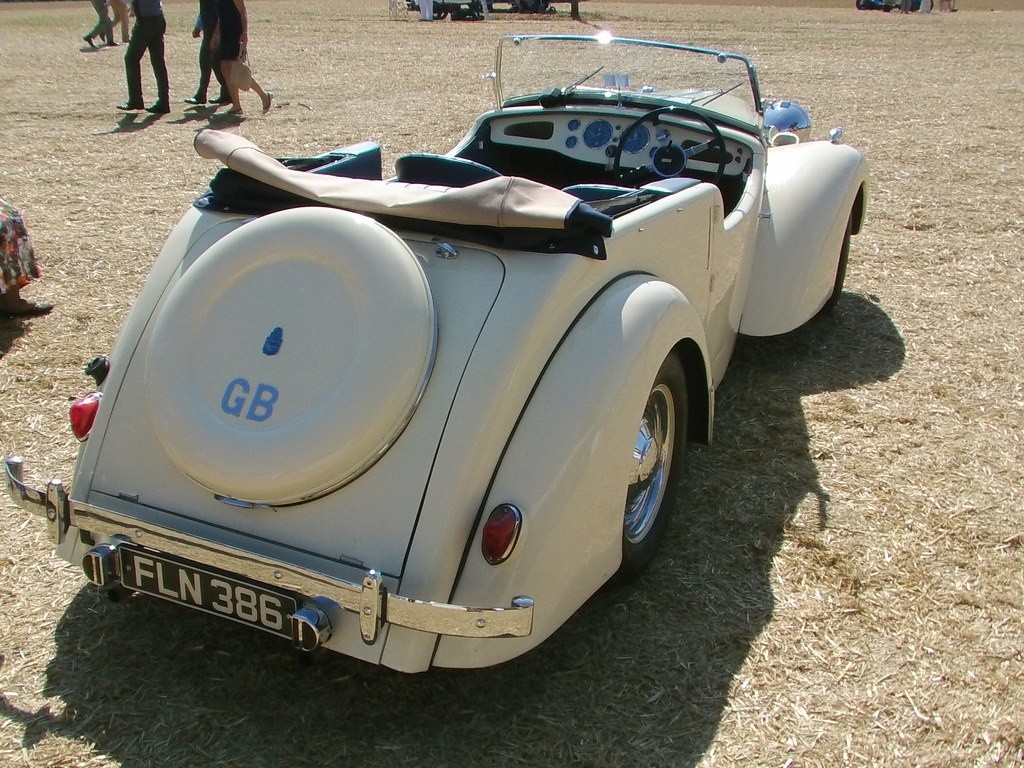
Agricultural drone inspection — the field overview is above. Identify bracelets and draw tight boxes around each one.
[242,31,250,34]
[213,34,220,38]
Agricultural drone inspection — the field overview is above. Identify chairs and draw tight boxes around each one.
[395,153,502,188]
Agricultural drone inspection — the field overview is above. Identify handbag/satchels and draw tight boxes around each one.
[230,42,252,91]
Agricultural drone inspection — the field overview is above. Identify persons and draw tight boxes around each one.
[116,0,170,112]
[82,0,134,47]
[0,197,54,315]
[900,0,958,14]
[417,0,433,21]
[184,0,273,115]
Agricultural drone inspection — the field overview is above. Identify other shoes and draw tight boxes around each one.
[0,302,54,316]
[107,43,119,46]
[227,109,243,114]
[99,33,105,42]
[263,92,273,115]
[83,35,96,48]
[122,40,131,43]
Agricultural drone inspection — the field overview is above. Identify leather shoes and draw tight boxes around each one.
[117,103,144,110]
[208,97,232,103]
[184,98,206,104]
[145,105,170,113]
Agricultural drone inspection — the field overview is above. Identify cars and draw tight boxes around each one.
[5,33,867,675]
